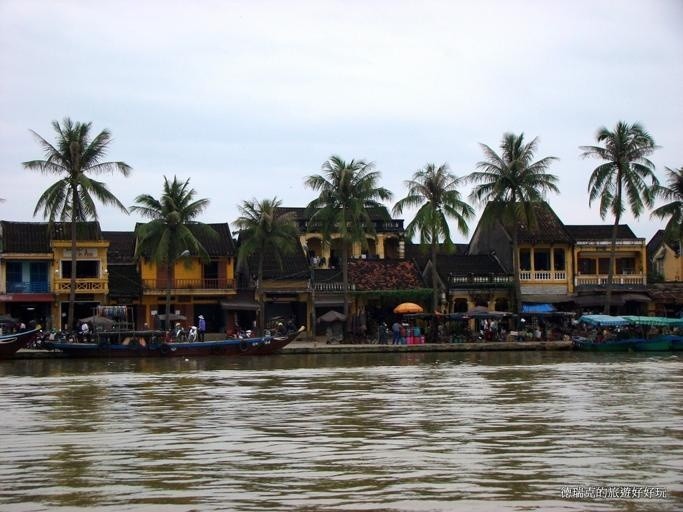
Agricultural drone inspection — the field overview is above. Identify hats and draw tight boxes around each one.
[198,315,204,320]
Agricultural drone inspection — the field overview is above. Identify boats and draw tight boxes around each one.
[43,323,308,358]
[0,322,45,358]
[566,312,682,354]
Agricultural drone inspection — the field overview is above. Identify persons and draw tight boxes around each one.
[15,314,297,344]
[563,308,682,343]
[378,315,546,345]
[307,254,379,270]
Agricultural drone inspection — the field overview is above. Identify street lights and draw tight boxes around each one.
[163,248,191,330]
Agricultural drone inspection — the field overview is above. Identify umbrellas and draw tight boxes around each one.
[392,302,423,315]
[315,310,348,324]
[430,306,512,321]
